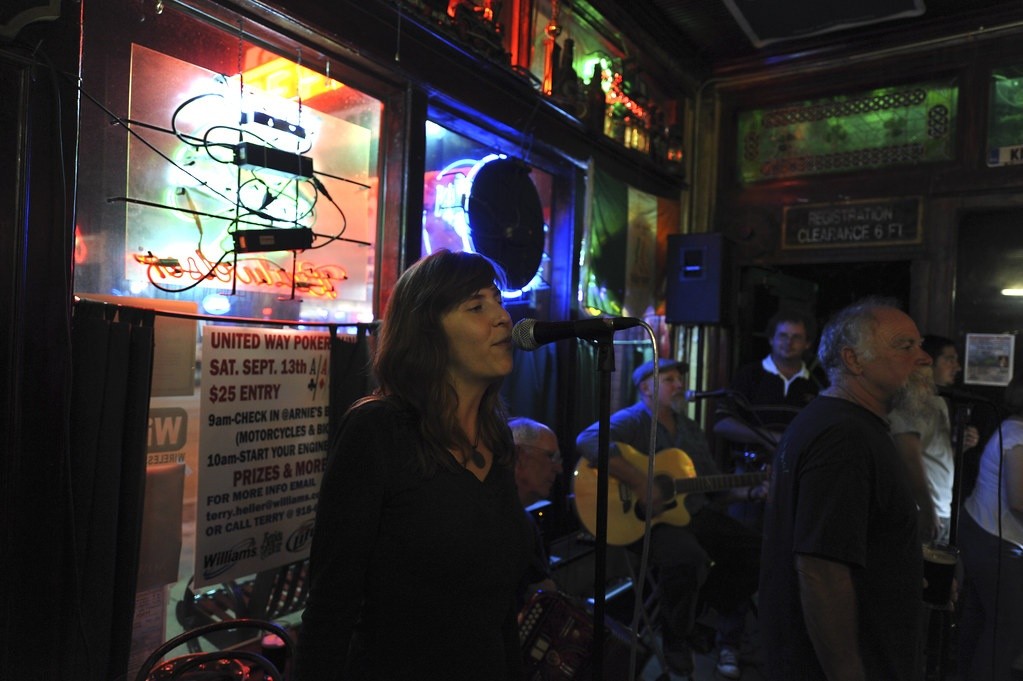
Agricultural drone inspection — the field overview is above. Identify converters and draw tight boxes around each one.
[234,142,313,180]
[232,228,312,254]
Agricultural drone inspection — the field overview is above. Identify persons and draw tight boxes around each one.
[885,334,1023,681]
[293,249,562,681]
[710,307,825,677]
[759,295,924,681]
[576,358,762,676]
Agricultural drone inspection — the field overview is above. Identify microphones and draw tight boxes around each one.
[684,388,727,402]
[508,315,640,352]
[937,383,995,407]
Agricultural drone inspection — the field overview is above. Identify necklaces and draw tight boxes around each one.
[472,433,486,469]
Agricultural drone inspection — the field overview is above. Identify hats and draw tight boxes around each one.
[633,359,690,387]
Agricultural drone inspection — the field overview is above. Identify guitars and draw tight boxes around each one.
[566,439,773,544]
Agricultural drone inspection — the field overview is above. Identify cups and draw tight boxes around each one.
[923,541,960,601]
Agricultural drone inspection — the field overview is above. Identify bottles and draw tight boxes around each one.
[557,38,669,163]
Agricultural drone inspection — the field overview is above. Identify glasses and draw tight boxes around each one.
[521,442,563,469]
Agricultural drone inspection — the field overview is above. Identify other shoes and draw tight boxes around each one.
[662,632,692,676]
[717,648,739,677]
[690,625,714,654]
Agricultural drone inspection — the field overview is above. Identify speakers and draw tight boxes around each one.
[664,232,722,325]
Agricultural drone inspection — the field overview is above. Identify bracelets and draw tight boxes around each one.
[748,487,755,503]
